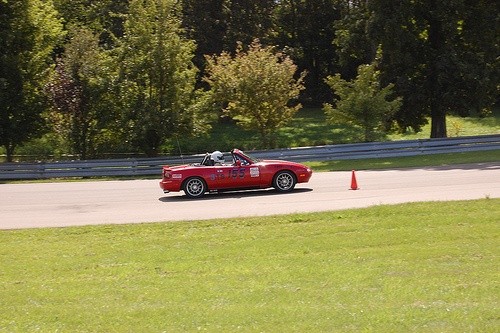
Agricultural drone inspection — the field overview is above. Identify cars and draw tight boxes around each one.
[159,148,312,199]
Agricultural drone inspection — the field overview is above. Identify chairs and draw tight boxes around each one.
[201,152,214,165]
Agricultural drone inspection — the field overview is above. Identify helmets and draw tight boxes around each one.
[211,150,225,163]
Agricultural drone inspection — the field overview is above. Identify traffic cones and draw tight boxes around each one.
[349,170,360,190]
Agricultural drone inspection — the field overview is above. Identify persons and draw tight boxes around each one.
[211,151,226,165]
